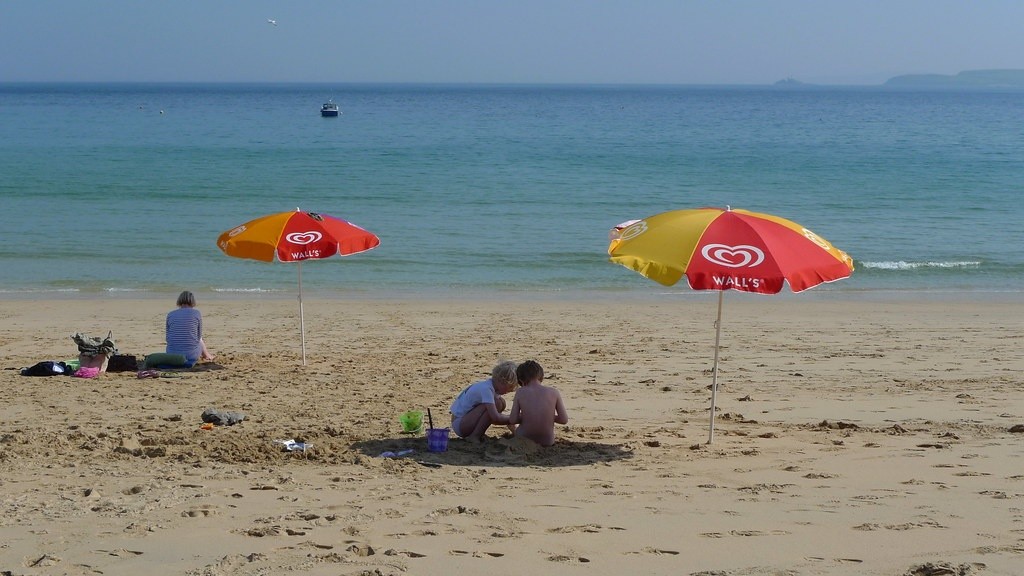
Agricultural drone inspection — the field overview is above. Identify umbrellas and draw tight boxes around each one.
[607,205,854,443]
[217,207,381,365]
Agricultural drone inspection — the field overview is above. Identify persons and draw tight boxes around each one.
[449,360,518,444]
[166,291,212,360]
[509,360,568,446]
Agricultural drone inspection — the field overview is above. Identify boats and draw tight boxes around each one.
[321,99,338,116]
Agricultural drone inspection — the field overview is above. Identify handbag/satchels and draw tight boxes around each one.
[106,354,137,372]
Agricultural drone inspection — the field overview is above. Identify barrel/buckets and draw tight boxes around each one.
[400,411,424,434]
[426,427,451,452]
